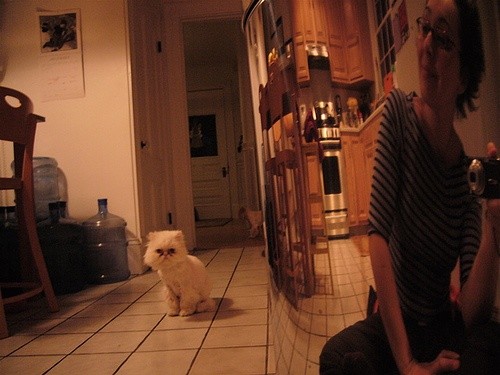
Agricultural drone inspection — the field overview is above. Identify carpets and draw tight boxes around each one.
[352,234,370,256]
[195,219,230,228]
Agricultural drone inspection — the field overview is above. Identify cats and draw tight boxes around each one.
[238,205,262,238]
[143,229,215,317]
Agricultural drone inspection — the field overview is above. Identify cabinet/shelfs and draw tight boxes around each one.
[303,148,323,238]
[341,109,383,236]
[288,0,375,88]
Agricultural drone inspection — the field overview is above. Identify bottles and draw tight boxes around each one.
[35,200,85,296]
[10,155,59,224]
[82,197,132,284]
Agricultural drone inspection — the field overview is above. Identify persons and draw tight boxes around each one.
[320,0,500,375]
[53,19,68,43]
[40,22,58,53]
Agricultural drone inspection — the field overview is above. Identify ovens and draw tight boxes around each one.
[321,139,349,236]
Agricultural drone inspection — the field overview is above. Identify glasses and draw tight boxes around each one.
[416,16,460,53]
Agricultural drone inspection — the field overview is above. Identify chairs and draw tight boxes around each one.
[258,64,334,312]
[0,86,60,340]
